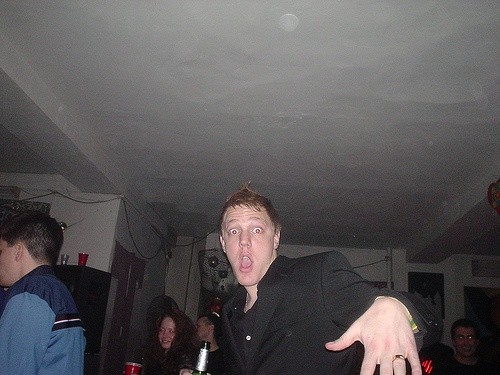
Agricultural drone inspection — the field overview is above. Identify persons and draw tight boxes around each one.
[220,179,443,375]
[431,318,500,375]
[143,309,225,375]
[0,212,86,375]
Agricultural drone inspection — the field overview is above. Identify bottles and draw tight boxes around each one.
[191,340,211,375]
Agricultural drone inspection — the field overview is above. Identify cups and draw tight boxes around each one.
[61,255,69,265]
[124,362,143,375]
[79,252,89,266]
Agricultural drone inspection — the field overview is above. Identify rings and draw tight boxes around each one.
[392,355,405,362]
